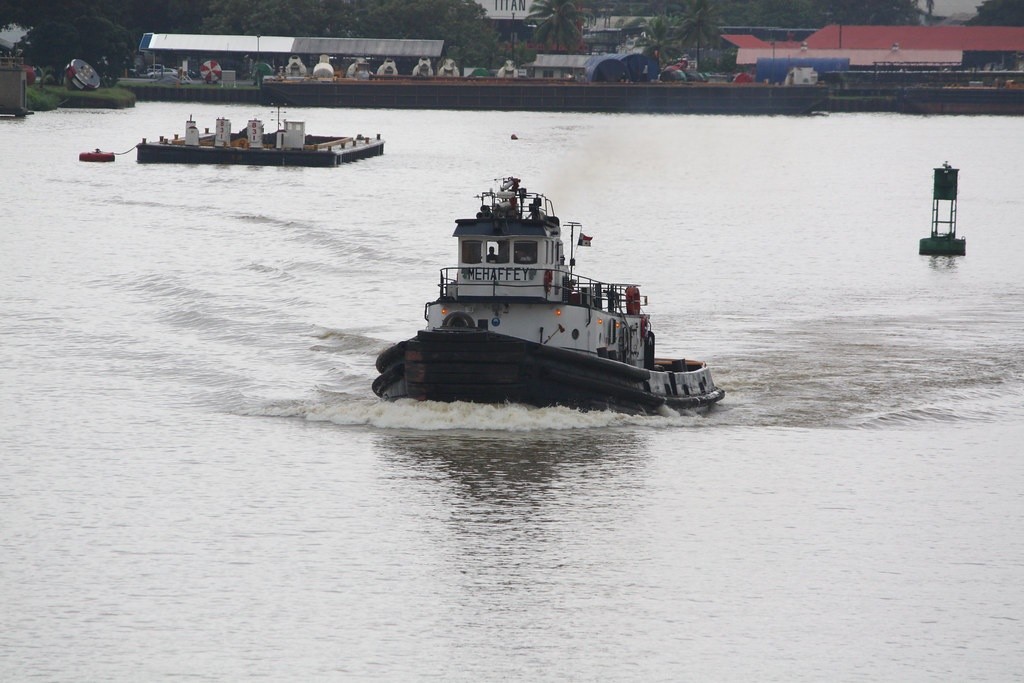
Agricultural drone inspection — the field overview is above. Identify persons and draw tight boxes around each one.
[487,246,499,264]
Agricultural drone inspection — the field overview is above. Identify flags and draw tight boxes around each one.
[578,232,593,246]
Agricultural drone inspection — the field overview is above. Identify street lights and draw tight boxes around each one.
[511,11,516,60]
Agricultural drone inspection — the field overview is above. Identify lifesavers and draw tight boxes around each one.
[626,287,640,315]
[641,313,649,337]
[544,271,553,293]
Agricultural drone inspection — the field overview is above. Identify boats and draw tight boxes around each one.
[371,176,725,416]
[436,60,460,76]
[377,58,399,75]
[412,57,434,76]
[495,60,520,78]
[313,55,335,78]
[285,56,307,76]
[345,58,374,78]
